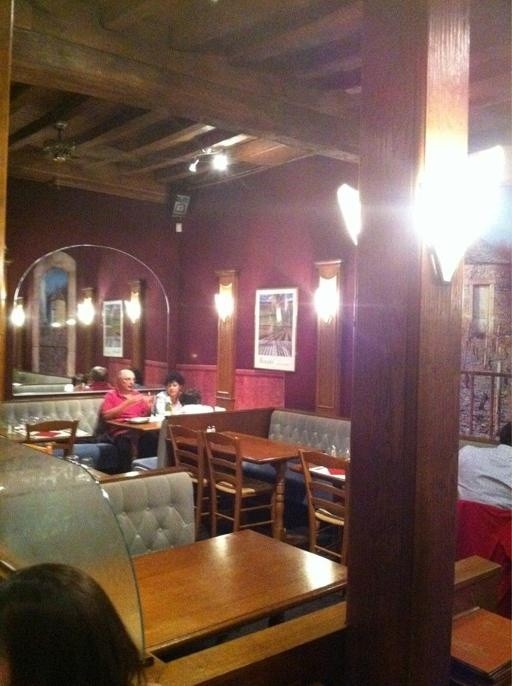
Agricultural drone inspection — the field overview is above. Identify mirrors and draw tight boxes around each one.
[11,246,171,402]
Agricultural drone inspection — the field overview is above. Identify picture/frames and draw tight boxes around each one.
[253,286,299,372]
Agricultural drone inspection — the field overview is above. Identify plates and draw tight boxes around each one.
[127,417,149,424]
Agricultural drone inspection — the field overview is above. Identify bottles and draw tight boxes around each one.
[207,426,216,433]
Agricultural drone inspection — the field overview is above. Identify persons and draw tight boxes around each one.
[65,373,89,392]
[1,562,140,685]
[153,376,182,416]
[458,421,512,512]
[268,294,289,357]
[88,366,114,390]
[177,388,213,415]
[101,368,156,459]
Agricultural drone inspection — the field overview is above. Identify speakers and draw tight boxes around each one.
[165,193,191,220]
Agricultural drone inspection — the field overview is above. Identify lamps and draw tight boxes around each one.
[123,280,144,325]
[187,145,239,174]
[314,261,341,326]
[9,297,26,328]
[75,288,96,326]
[214,271,239,323]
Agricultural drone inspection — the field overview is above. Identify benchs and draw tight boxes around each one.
[12,369,73,393]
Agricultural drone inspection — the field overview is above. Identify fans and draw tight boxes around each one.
[8,119,144,164]
